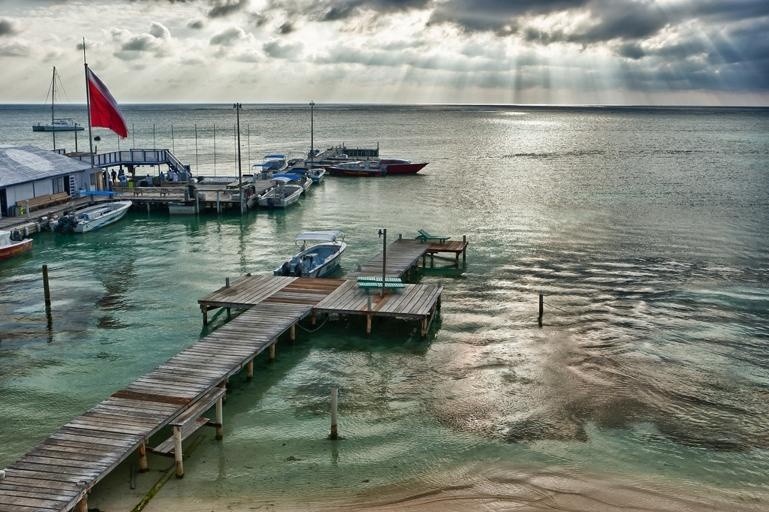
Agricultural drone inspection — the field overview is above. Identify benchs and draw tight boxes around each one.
[14,195,54,214]
[225,176,248,194]
[358,274,402,281]
[51,191,71,205]
[358,283,405,294]
[121,187,168,198]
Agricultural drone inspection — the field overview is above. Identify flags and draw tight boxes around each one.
[86,67,127,140]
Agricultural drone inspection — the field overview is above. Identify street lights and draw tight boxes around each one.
[378,227,387,298]
[308,102,317,160]
[234,102,250,202]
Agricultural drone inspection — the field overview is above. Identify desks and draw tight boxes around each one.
[79,191,117,199]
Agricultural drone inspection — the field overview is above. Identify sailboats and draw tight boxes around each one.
[33,66,85,132]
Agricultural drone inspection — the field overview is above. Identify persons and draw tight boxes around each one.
[281,239,317,276]
[331,233,337,243]
[103,168,173,187]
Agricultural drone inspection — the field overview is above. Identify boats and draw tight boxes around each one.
[50,199,133,234]
[327,160,389,177]
[304,167,328,184]
[0,229,34,260]
[242,154,305,183]
[256,183,304,210]
[287,175,312,195]
[312,158,430,175]
[273,230,347,280]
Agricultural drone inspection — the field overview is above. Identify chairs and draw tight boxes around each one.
[417,228,452,246]
